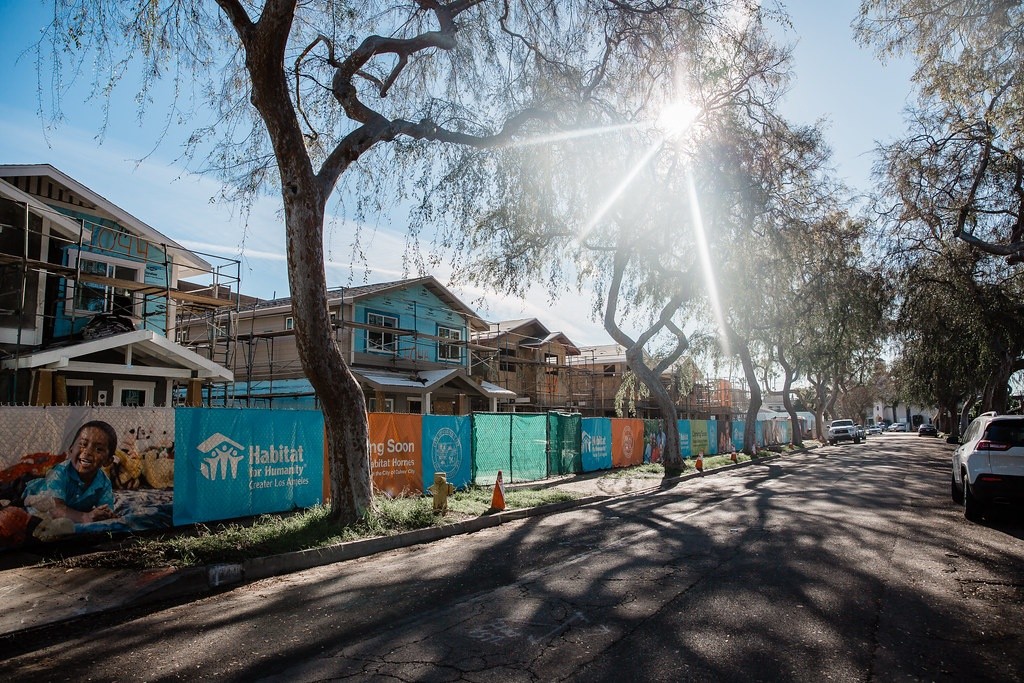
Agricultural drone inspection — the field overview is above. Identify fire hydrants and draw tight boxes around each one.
[427,471,454,516]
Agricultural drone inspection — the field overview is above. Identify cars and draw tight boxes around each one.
[856,424,884,436]
[918,423,937,437]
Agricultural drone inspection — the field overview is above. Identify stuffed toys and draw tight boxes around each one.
[2,426,176,541]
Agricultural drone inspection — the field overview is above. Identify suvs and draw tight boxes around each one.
[826,419,861,445]
[945,411,1024,522]
[888,422,906,432]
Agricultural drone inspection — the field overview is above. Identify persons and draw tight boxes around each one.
[643,419,667,466]
[2,419,118,524]
[718,421,732,453]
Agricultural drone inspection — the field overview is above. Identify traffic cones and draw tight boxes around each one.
[750,442,757,458]
[788,438,793,449]
[485,470,510,512]
[694,447,704,471]
[729,445,738,461]
